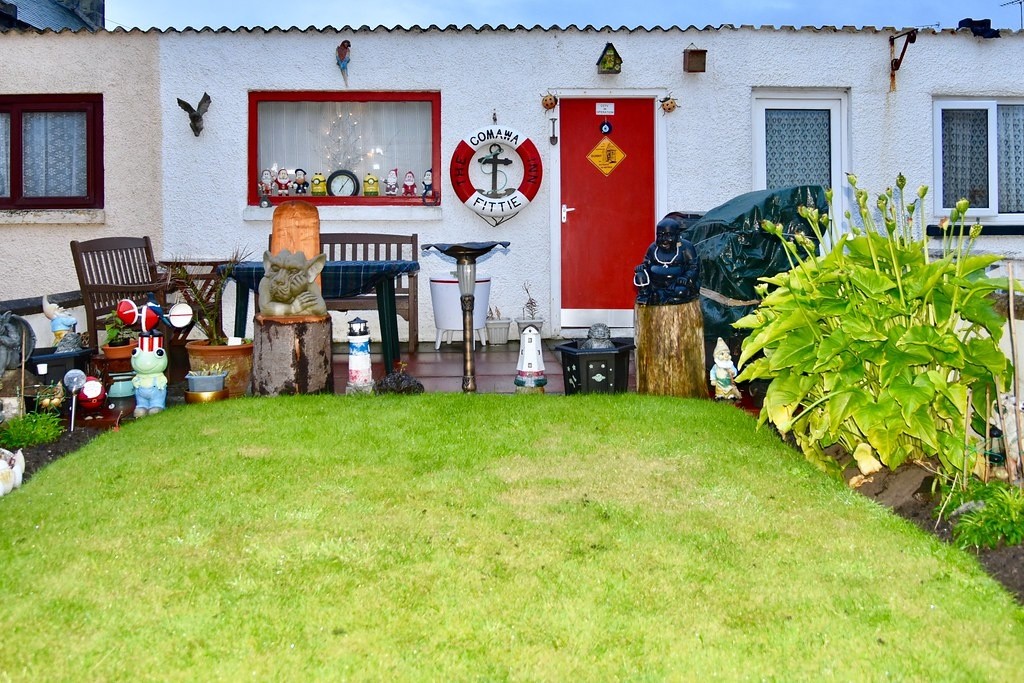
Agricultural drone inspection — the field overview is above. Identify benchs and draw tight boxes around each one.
[70,236,231,385]
[269,233,419,354]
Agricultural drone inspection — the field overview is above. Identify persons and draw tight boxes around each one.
[633,218,700,307]
[380,168,399,196]
[258,169,275,196]
[293,168,309,196]
[44,304,77,346]
[78,376,106,420]
[710,337,742,399]
[273,168,294,196]
[422,169,432,195]
[402,171,417,197]
[0,306,20,377]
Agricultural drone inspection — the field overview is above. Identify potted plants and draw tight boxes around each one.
[185,359,230,392]
[486,305,513,344]
[139,241,261,396]
[101,310,133,347]
[514,280,545,339]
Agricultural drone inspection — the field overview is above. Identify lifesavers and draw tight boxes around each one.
[449,123,544,218]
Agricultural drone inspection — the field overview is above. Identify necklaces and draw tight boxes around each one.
[654,244,679,267]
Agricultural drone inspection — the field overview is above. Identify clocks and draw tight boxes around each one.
[326,170,360,196]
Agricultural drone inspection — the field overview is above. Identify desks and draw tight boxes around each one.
[217,259,422,376]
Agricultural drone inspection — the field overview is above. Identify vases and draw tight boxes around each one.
[18,346,96,415]
[554,338,637,396]
[101,340,138,358]
[184,386,229,404]
